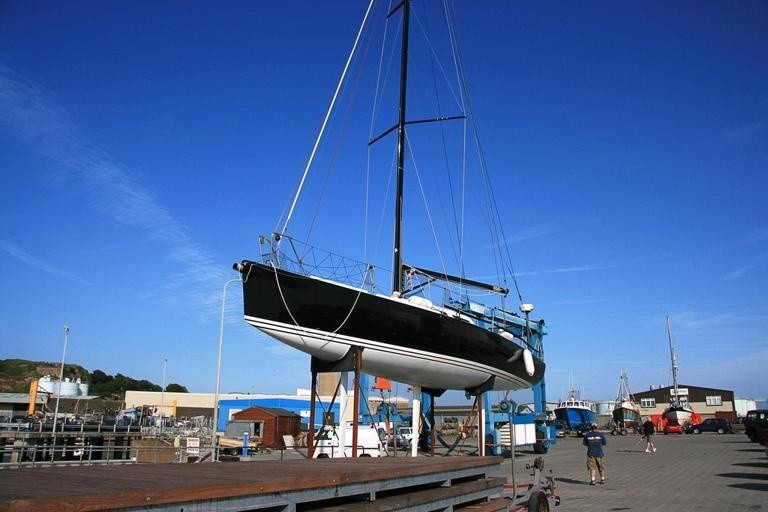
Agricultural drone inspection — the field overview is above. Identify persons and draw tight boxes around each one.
[582,422,607,485]
[642,416,657,453]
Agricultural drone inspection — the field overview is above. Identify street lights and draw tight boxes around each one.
[52,326,70,437]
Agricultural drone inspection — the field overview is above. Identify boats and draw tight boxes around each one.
[554,391,597,431]
[613,375,640,429]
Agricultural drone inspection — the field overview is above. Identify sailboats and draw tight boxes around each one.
[233,0,546,390]
[663,315,694,426]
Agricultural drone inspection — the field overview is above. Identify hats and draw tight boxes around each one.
[591,423,597,428]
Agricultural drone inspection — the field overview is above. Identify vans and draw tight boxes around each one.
[313,425,412,459]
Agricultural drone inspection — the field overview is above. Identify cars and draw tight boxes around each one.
[693,417,730,434]
[577,424,592,437]
[663,421,684,435]
[552,424,564,438]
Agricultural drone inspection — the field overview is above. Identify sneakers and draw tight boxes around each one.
[645,449,651,453]
[653,448,657,452]
[601,479,605,484]
[589,480,596,485]
